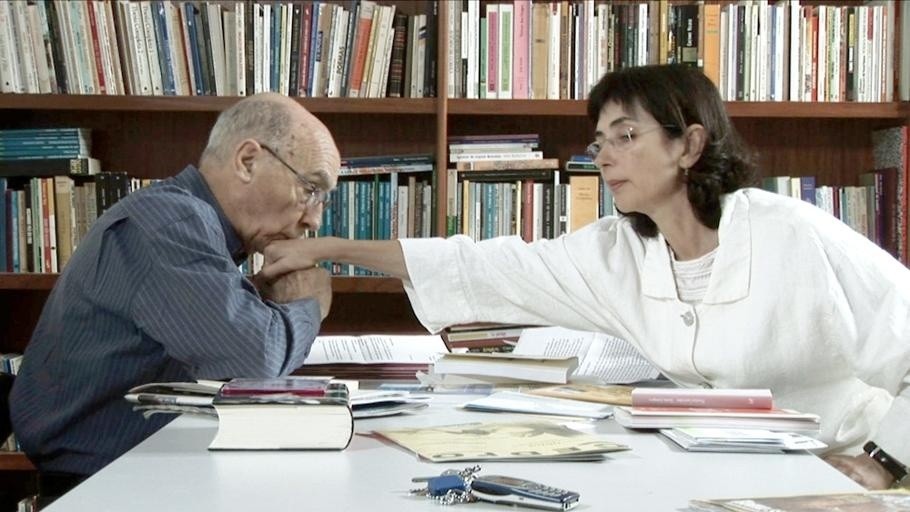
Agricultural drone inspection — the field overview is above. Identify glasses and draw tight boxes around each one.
[253,140,332,211]
[587,119,683,170]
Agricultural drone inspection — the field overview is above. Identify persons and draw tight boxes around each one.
[10,93,342,481]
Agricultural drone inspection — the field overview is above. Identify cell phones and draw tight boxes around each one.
[471,473,580,512]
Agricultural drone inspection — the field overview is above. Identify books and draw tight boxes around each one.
[614,383,822,432]
[434,352,579,386]
[1,1,437,277]
[18,493,40,512]
[1,352,24,378]
[445,1,904,264]
[208,377,355,453]
[442,325,548,354]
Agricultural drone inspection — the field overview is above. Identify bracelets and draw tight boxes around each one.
[863,439,907,482]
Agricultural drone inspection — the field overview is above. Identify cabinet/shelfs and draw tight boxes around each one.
[0,0,910,512]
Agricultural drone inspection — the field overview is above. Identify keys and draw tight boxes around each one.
[406,466,481,505]
[260,63,909,491]
